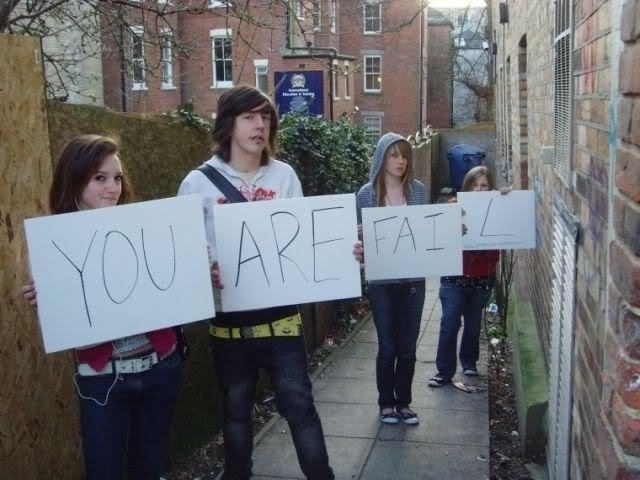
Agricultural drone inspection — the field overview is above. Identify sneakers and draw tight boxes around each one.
[378,408,401,424]
[427,372,454,386]
[395,405,420,425]
[459,359,478,375]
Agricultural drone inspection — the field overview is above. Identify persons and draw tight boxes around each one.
[355,132,468,424]
[22,135,212,478]
[428,165,513,387]
[173,85,365,480]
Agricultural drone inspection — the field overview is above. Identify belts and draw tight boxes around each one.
[76,342,178,377]
[209,313,304,339]
[367,278,426,284]
